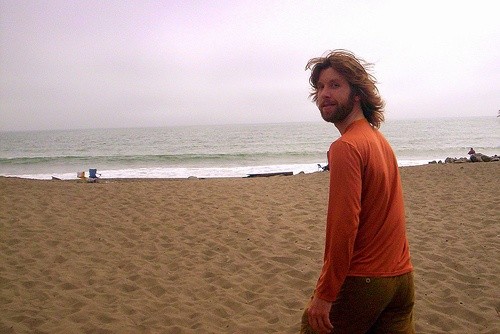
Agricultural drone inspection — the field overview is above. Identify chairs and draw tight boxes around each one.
[76,171,88,183]
[88,169,102,183]
[317,164,325,172]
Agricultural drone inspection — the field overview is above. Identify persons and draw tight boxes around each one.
[300,48,415,334]
[468,148,475,154]
